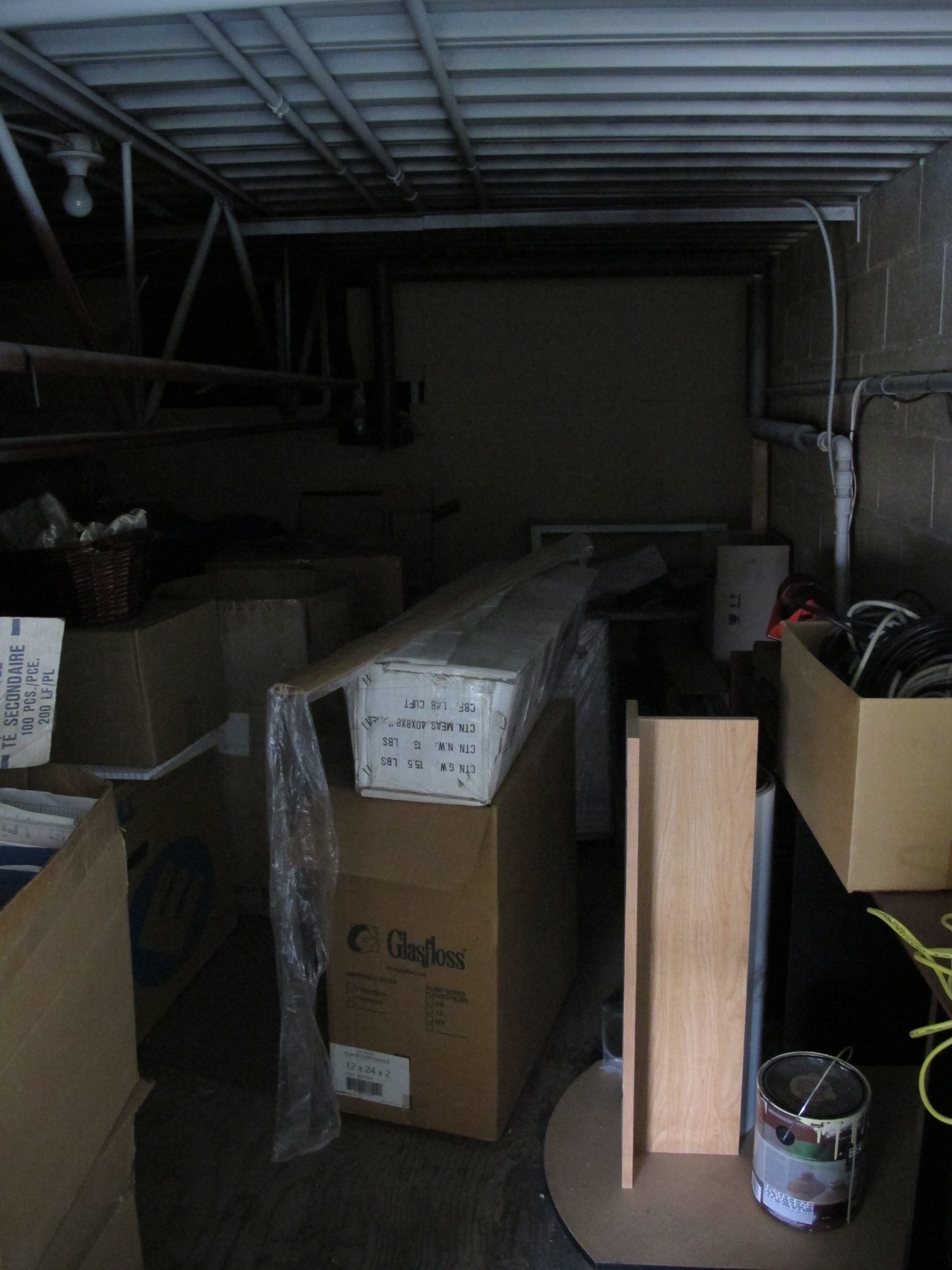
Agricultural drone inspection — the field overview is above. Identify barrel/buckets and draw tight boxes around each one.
[751,1047,871,1232]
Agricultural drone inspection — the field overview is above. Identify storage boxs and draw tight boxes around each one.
[781,621,952,893]
[0,556,585,1270]
